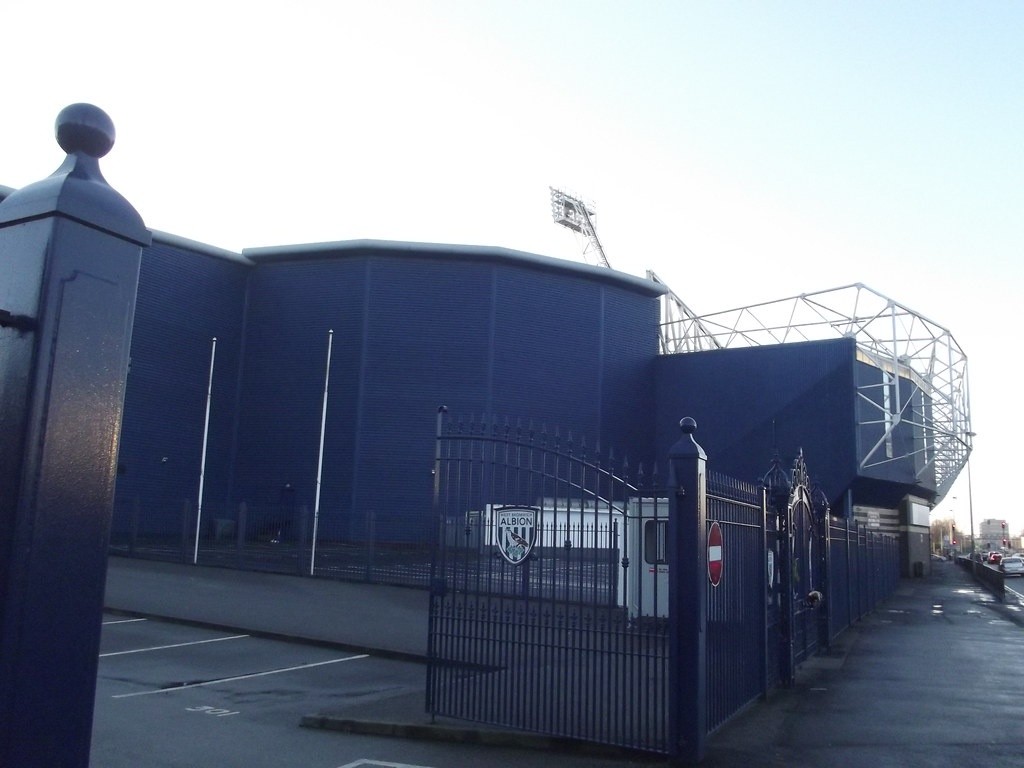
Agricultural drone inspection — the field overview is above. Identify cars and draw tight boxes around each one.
[969,549,1024,577]
[931,553,946,563]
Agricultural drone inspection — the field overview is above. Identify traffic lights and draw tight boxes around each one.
[953,539,957,544]
[1003,539,1007,545]
[1002,522,1005,528]
[952,524,955,528]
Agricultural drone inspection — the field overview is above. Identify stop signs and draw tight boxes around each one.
[707,522,723,587]
